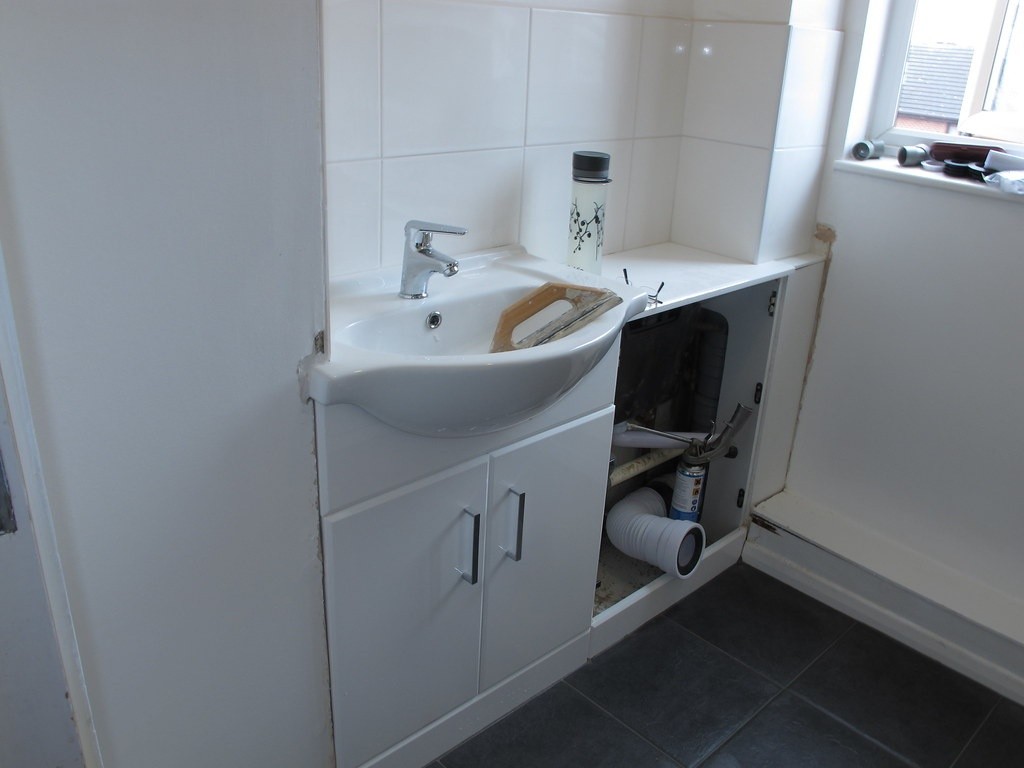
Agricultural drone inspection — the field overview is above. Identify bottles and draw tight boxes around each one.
[568,150,613,275]
[670,464,706,523]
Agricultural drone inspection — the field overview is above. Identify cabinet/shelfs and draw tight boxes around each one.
[320,278,784,768]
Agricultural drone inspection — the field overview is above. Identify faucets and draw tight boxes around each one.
[397,219,467,300]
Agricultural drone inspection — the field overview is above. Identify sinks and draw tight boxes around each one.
[330,242,649,440]
